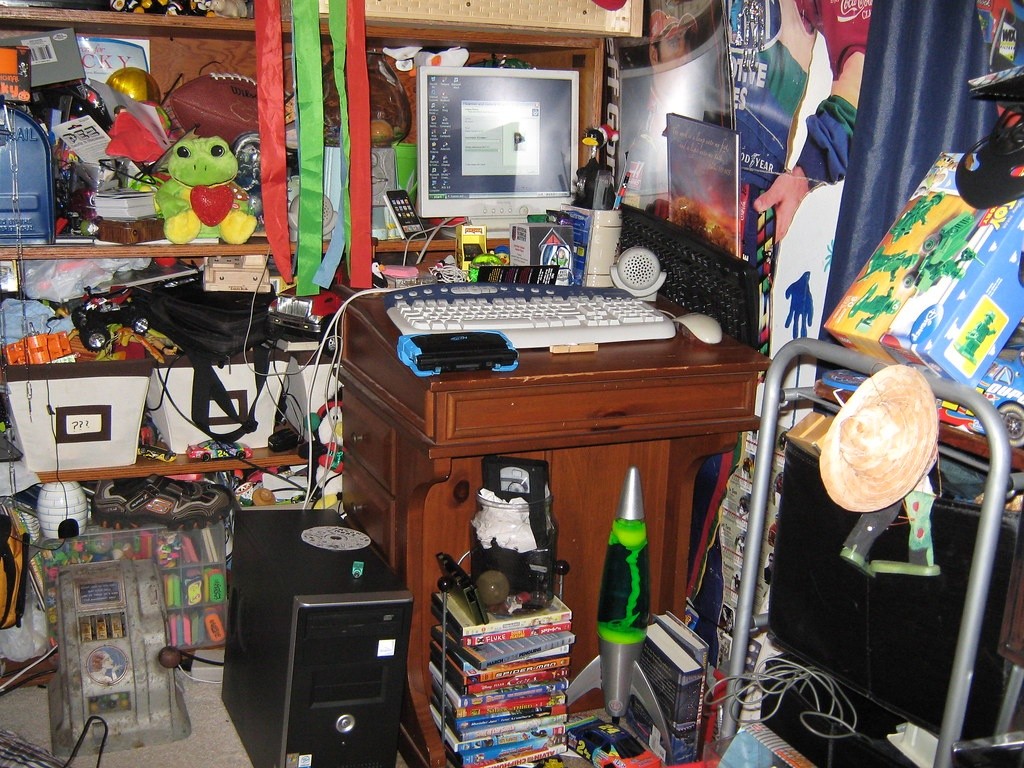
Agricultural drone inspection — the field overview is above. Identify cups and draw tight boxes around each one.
[573,206,622,287]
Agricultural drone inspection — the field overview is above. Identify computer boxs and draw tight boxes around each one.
[222,509,414,768]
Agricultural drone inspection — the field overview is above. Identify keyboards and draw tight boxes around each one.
[617,203,759,351]
[384,281,676,349]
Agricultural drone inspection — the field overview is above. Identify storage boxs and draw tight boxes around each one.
[147,350,291,455]
[0,28,86,103]
[282,350,343,444]
[3,358,153,472]
[47,518,229,671]
[713,151,1024,768]
[510,223,574,286]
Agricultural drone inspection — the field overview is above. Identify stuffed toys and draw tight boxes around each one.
[573,124,619,210]
[156,134,257,244]
[297,386,343,507]
[493,246,510,266]
[372,259,390,289]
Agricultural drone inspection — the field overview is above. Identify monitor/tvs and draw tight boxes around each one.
[416,65,579,238]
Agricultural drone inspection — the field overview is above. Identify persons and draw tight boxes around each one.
[553,249,569,267]
[718,429,789,675]
[726,0,871,357]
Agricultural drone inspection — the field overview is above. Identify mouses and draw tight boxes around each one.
[673,312,722,343]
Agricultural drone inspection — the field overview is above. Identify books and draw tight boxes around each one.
[626,611,709,767]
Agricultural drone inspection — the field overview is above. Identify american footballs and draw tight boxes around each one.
[169,72,259,145]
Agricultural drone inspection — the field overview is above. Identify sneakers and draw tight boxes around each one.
[91,476,233,531]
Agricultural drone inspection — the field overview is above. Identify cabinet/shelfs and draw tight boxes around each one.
[0,9,602,691]
[318,1,644,37]
[330,282,773,768]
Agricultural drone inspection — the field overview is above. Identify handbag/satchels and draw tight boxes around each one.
[0,514,29,630]
[148,281,278,445]
